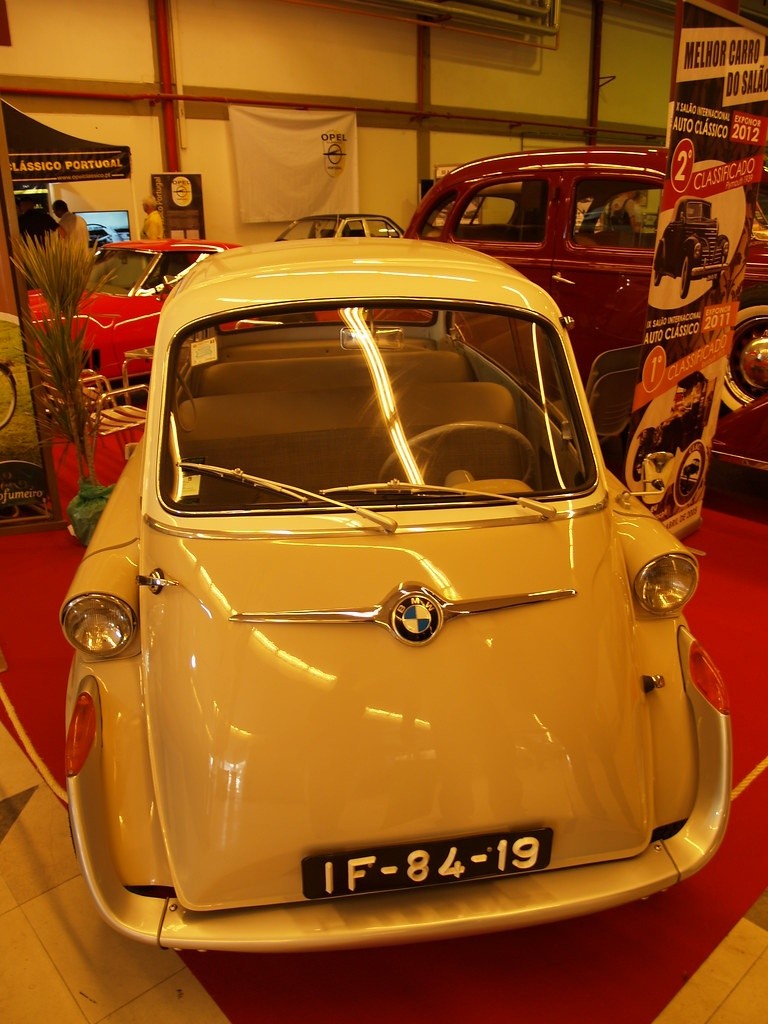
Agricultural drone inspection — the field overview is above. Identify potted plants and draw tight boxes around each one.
[6,225,115,547]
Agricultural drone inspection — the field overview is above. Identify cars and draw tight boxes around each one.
[405,146,767,494]
[276,213,406,244]
[54,233,731,953]
[81,224,113,247]
[653,195,731,300]
[26,239,272,416]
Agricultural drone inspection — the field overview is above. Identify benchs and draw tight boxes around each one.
[166,381,518,507]
[207,336,436,365]
[194,349,474,395]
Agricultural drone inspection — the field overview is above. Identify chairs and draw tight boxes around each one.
[80,372,148,489]
[546,342,642,459]
[320,229,334,237]
[101,254,149,288]
[611,209,655,246]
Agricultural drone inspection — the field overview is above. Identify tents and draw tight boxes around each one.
[1,99,140,246]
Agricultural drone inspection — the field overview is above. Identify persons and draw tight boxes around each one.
[53,201,91,250]
[141,195,164,241]
[18,198,67,248]
[572,188,645,233]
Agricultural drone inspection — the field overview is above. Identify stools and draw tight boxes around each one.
[122,337,192,406]
